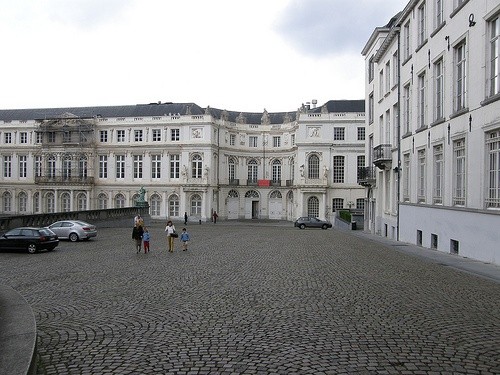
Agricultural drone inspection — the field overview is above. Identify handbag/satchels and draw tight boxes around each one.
[170,232,178,238]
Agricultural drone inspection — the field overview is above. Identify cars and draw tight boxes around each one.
[45,220,97,242]
[0,227,59,254]
[294,216,332,230]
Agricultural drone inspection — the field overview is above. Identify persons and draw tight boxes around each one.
[141,227,151,254]
[165,221,176,252]
[213,211,218,224]
[184,212,188,225]
[132,214,144,255]
[180,228,190,251]
